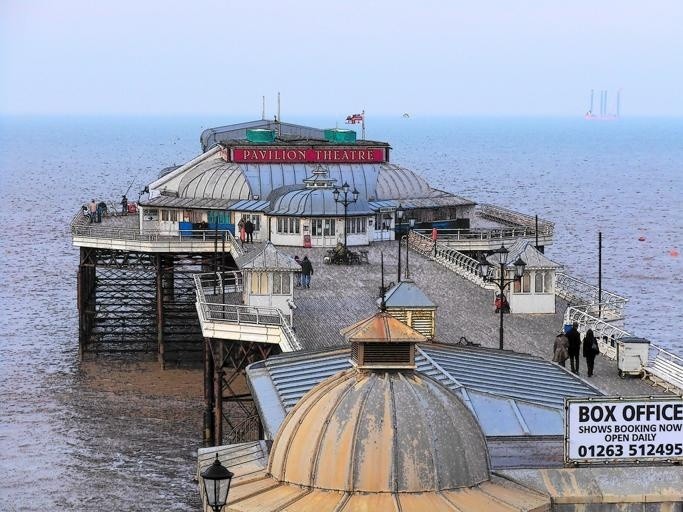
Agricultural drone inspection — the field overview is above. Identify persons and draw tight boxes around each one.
[121,195,128,216]
[583,329,598,377]
[82,198,106,224]
[553,328,569,367]
[199,221,206,229]
[301,256,313,288]
[294,255,304,287]
[566,322,580,375]
[238,218,245,243]
[334,243,344,263]
[245,218,254,243]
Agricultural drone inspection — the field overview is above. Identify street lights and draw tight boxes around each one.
[478,242,527,350]
[332,181,359,251]
[383,203,417,282]
[200,452,234,512]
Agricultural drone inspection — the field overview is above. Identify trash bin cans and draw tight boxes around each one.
[614,335,650,380]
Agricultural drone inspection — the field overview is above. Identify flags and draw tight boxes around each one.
[346,113,362,124]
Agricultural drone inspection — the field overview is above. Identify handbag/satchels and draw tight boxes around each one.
[592,343,599,354]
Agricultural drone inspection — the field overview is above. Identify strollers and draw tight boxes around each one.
[494,291,510,313]
